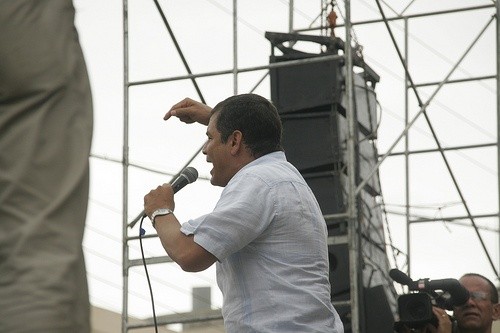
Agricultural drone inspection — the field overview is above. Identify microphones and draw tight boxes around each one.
[143,167,199,216]
[389,269,412,286]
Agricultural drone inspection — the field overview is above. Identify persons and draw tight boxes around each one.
[142,93,346,332]
[433,272,500,332]
[0,0,93,333]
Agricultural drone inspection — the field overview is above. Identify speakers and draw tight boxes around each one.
[268,52,402,333]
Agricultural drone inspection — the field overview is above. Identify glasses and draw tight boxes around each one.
[469,292,493,305]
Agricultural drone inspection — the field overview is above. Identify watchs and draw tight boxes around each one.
[151,208,173,226]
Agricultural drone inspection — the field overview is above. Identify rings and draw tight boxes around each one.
[442,314,445,317]
